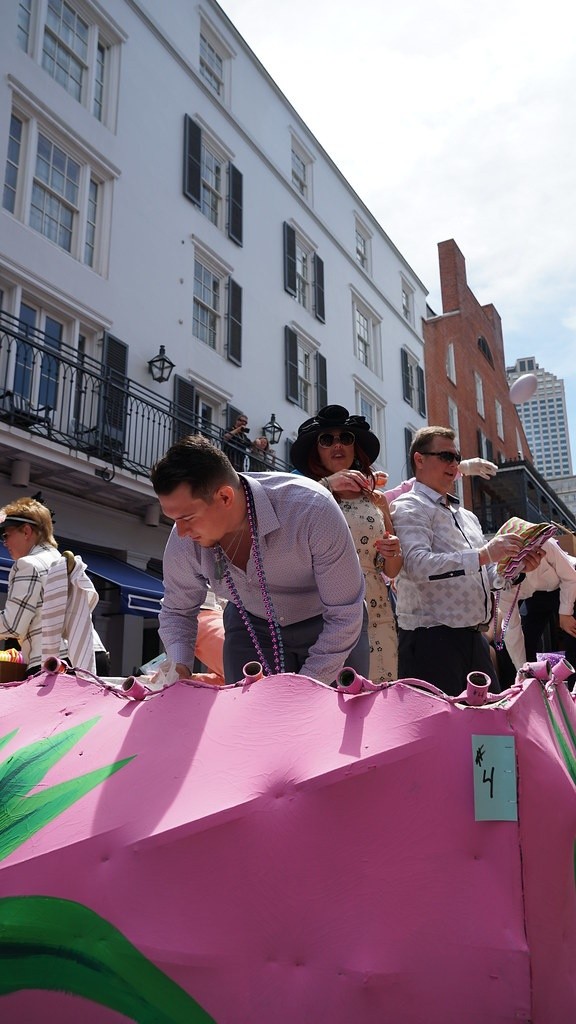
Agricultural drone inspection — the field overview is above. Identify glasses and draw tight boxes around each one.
[420,451,461,465]
[316,431,355,449]
[238,419,247,424]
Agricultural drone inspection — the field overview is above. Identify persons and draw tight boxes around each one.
[389,425,546,707]
[221,415,248,472]
[291,404,404,685]
[382,455,498,504]
[499,537,576,692]
[249,436,275,471]
[0,497,111,681]
[150,431,370,688]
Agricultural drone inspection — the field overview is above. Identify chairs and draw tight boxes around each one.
[0,389,54,441]
[73,420,119,467]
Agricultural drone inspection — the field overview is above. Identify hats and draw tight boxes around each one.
[493,516,558,582]
[290,405,380,482]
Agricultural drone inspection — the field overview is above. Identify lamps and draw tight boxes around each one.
[148,344,175,384]
[263,413,283,445]
